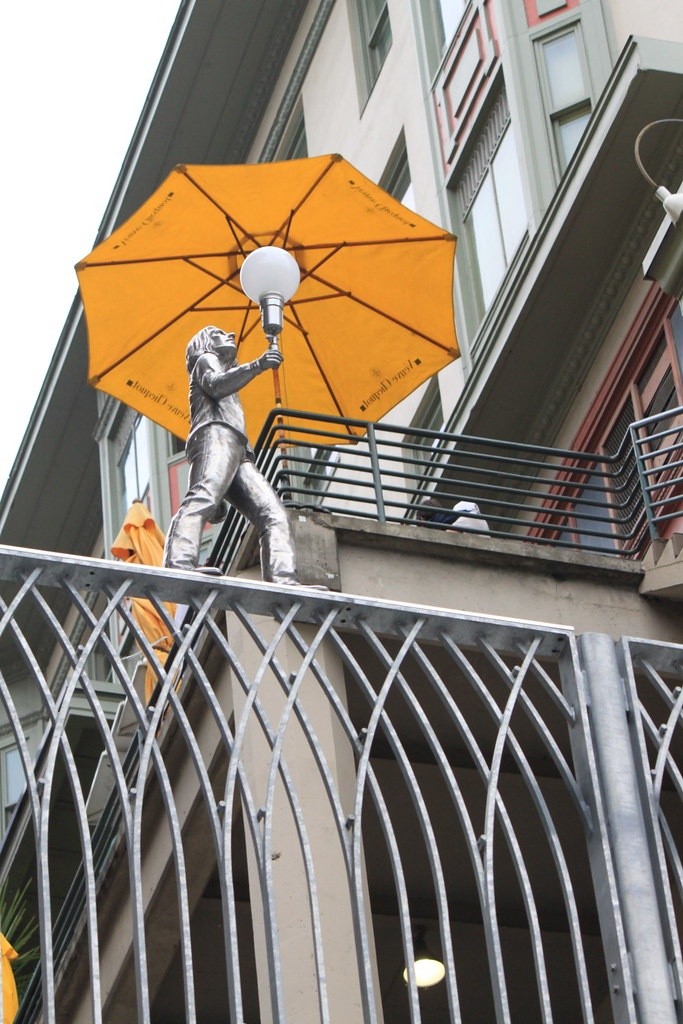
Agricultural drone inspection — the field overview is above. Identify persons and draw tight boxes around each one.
[164,326,332,592]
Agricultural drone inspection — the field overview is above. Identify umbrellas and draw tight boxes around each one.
[74,153,462,506]
[110,500,182,721]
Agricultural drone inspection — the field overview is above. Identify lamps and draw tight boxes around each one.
[403,925,446,989]
[633,119,683,298]
